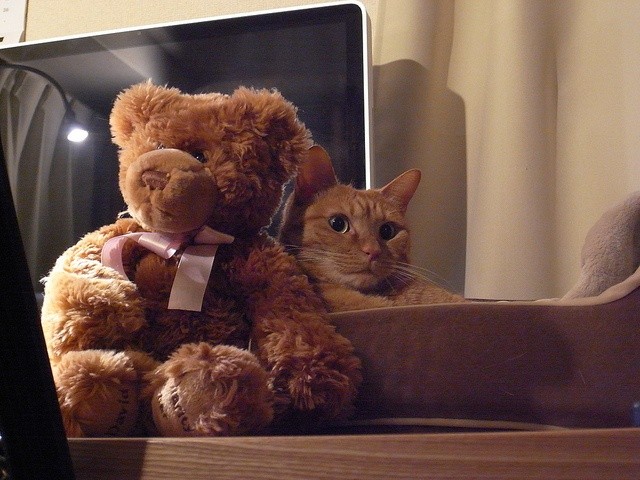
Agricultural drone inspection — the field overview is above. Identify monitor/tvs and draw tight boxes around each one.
[0,0,374,297]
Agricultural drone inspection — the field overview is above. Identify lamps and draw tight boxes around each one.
[65,110,90,143]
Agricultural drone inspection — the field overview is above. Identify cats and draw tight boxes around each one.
[279,145,468,311]
